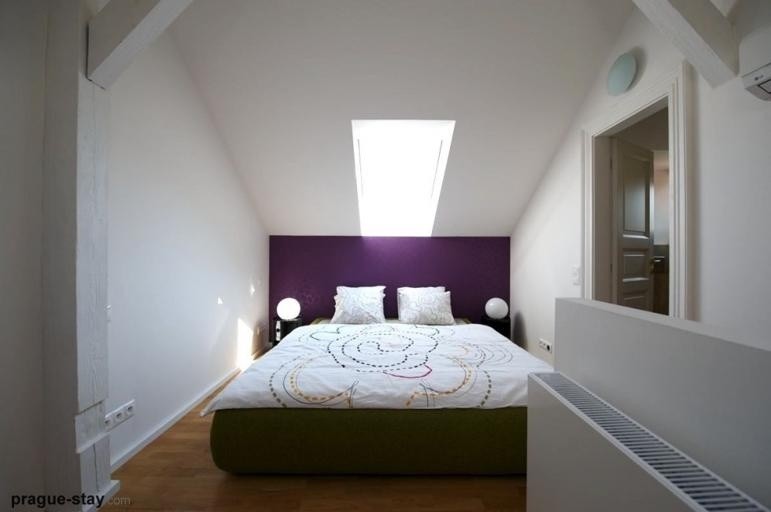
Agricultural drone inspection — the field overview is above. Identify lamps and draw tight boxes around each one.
[607,53,637,96]
[482,297,510,339]
[272,297,303,345]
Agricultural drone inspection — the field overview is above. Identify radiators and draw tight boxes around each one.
[525,297,771,512]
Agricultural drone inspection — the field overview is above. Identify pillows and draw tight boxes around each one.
[396,286,458,325]
[332,285,387,325]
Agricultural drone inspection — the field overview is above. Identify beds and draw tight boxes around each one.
[200,317,554,477]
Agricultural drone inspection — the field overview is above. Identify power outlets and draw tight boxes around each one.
[535,338,552,353]
[105,399,136,431]
